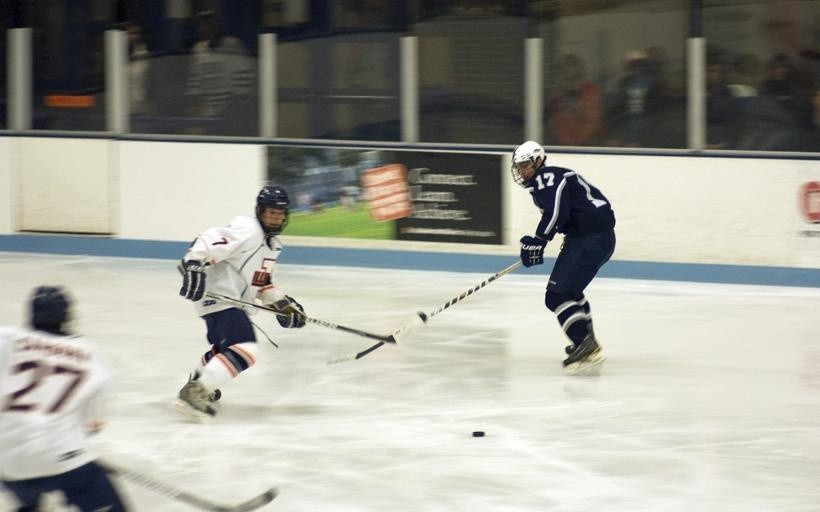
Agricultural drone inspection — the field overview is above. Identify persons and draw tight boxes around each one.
[614,50,665,144]
[546,54,605,144]
[175,184,307,418]
[121,17,154,117]
[707,38,820,149]
[0,283,128,512]
[183,9,258,136]
[510,140,616,368]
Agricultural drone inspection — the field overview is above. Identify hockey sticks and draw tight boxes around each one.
[204,291,427,345]
[327,261,522,363]
[99,457,280,512]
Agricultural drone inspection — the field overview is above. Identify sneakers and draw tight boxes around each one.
[179,376,221,416]
[563,334,600,367]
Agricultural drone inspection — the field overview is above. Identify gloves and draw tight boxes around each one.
[179,260,207,301]
[520,236,547,268]
[274,296,306,329]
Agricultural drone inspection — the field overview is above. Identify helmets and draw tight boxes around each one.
[512,140,545,169]
[31,285,70,332]
[256,185,288,237]
